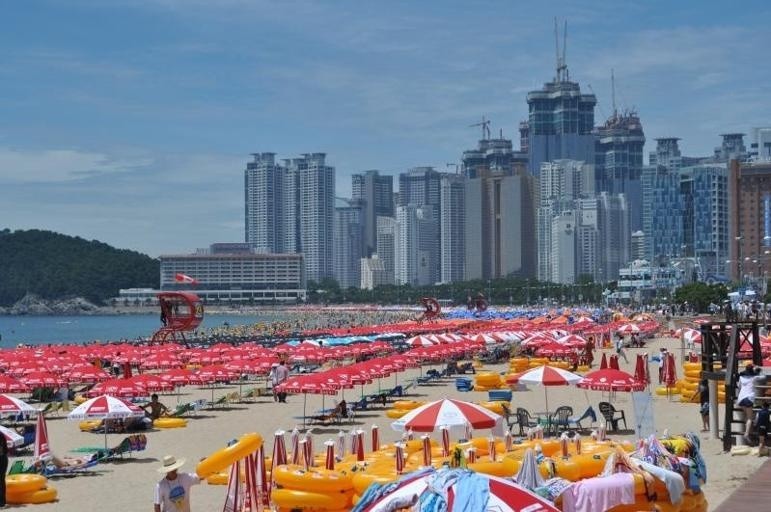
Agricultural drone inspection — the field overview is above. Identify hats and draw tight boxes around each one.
[157,454,187,473]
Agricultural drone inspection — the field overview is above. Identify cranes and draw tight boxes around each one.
[467,114,491,140]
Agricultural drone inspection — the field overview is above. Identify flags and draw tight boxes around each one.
[176,275,201,288]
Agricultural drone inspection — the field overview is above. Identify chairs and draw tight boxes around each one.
[502,402,627,438]
[27,331,208,420]
[90,435,147,463]
[293,413,350,425]
[320,399,381,416]
[362,382,416,406]
[208,331,286,411]
[286,331,535,386]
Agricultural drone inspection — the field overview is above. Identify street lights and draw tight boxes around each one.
[656,235,771,288]
[405,264,608,309]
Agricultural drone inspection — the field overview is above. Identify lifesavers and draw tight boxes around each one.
[262,453,302,482]
[4,474,58,503]
[510,435,635,465]
[472,357,591,392]
[386,400,425,418]
[656,438,691,461]
[80,420,101,431]
[656,362,727,403]
[312,453,339,467]
[339,435,458,509]
[197,432,263,481]
[480,400,511,415]
[468,454,521,477]
[277,506,349,512]
[552,445,614,482]
[153,417,185,427]
[207,469,246,484]
[555,470,707,512]
[272,488,350,510]
[468,436,507,457]
[273,462,354,492]
